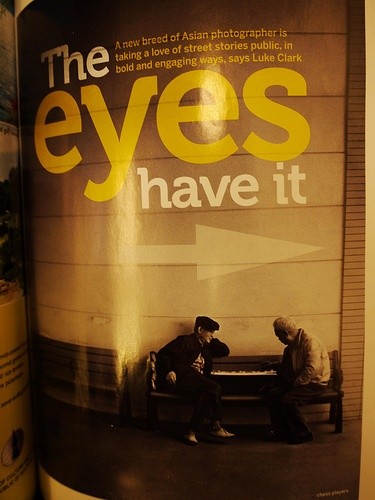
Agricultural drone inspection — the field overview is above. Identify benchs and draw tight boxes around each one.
[29,333,124,425]
[146,350,345,433]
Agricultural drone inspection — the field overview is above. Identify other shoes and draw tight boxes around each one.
[265,432,287,442]
[287,432,313,445]
[211,428,234,438]
[185,432,198,444]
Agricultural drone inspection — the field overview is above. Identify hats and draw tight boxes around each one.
[196,316,219,331]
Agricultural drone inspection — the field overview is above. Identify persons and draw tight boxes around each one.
[260,316,331,444]
[156,315,235,445]
